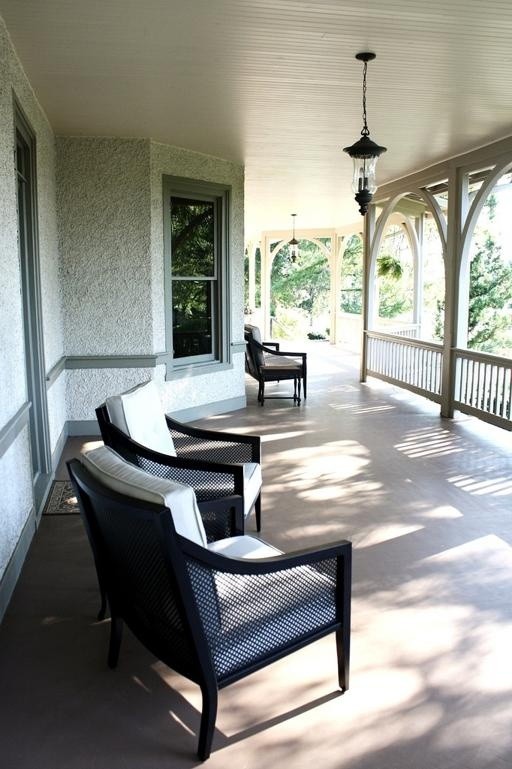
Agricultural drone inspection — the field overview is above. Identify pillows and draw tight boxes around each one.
[106,380,177,478]
[81,445,222,634]
[244,324,265,372]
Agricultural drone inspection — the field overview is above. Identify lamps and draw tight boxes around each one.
[343,51,387,215]
[287,214,300,263]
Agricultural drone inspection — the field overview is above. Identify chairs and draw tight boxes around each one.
[95,380,262,536]
[244,324,307,399]
[65,446,352,763]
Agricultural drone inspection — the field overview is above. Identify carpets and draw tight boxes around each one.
[42,480,80,516]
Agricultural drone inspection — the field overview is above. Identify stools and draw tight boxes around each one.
[258,366,301,407]
